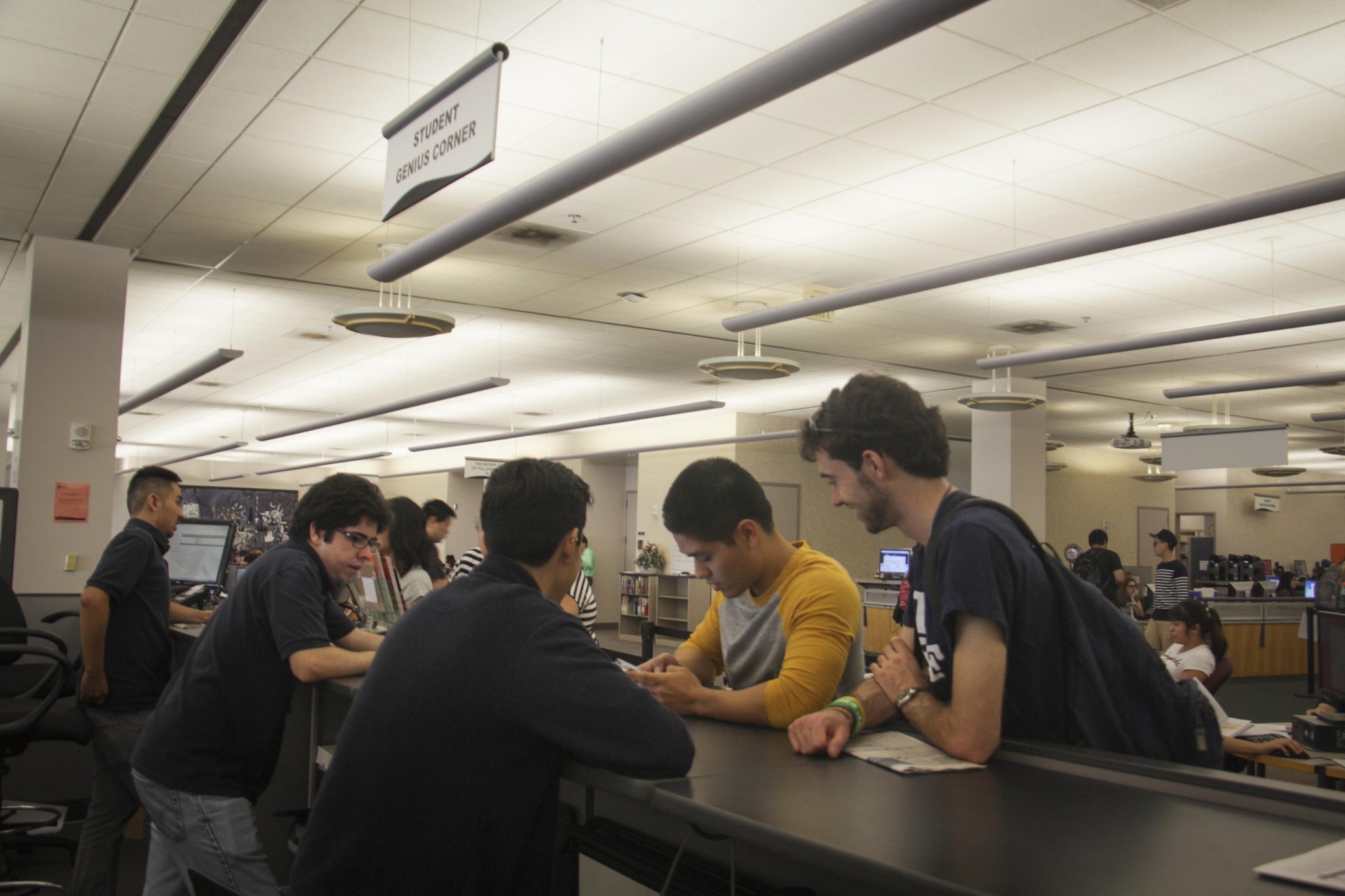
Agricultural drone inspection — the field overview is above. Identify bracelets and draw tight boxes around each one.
[823,694,867,736]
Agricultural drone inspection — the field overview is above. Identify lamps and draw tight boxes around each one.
[112,0,1345,495]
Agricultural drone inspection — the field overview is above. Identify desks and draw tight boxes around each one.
[1232,721,1345,791]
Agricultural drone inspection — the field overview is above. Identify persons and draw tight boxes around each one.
[71,466,221,896]
[1143,529,1190,657]
[129,473,386,896]
[1072,529,1125,613]
[787,373,1225,770]
[1151,558,1345,774]
[1120,578,1147,621]
[373,497,600,647]
[619,457,866,731]
[289,458,696,896]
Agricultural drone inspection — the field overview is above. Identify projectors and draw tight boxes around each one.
[1110,437,1152,450]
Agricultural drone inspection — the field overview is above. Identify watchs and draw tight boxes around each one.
[895,686,935,709]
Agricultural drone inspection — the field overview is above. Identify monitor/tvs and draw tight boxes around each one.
[160,518,234,589]
[879,548,911,575]
[1304,579,1317,598]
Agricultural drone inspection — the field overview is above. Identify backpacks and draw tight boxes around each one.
[930,504,1225,771]
[1073,548,1113,593]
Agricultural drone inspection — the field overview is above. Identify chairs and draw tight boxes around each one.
[0,576,85,896]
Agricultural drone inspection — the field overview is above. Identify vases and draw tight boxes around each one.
[636,563,662,574]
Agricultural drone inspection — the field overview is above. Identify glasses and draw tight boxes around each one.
[337,526,381,553]
[1153,541,1164,547]
[1127,584,1137,588]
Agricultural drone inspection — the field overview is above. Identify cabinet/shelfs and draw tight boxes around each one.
[618,571,657,643]
[655,573,711,647]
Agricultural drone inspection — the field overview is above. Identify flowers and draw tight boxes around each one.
[633,542,666,570]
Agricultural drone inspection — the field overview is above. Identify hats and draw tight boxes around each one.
[1149,529,1177,547]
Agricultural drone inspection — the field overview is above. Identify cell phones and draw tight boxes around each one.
[615,658,649,673]
